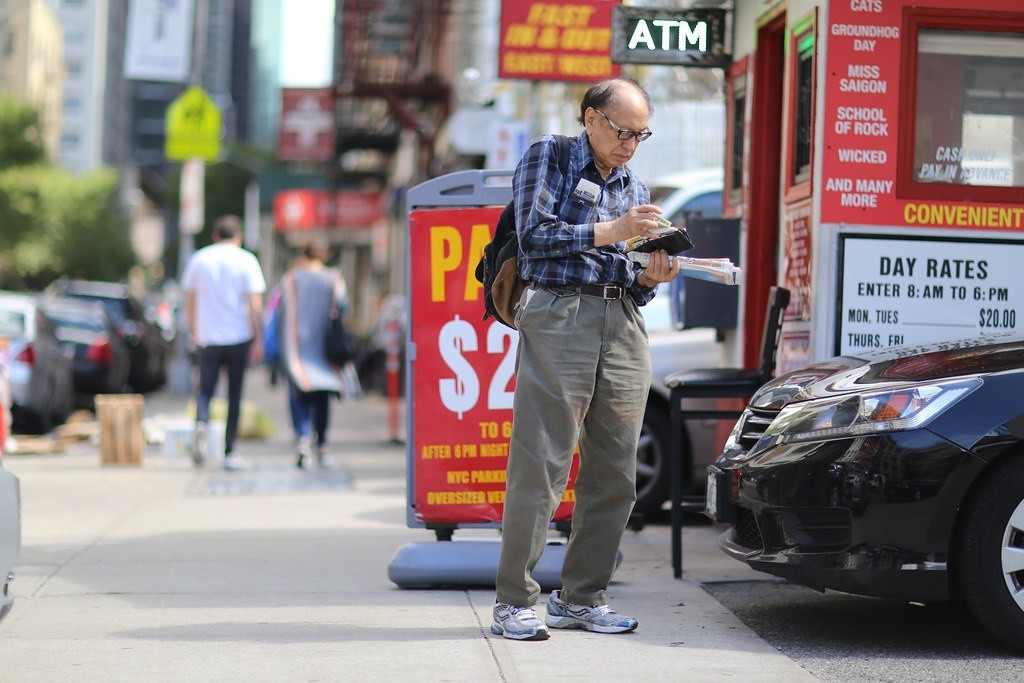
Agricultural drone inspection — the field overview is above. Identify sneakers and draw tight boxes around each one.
[544,590,638,634]
[490,597,550,640]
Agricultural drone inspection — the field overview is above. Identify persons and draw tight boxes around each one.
[182,214,366,471]
[475,79,680,641]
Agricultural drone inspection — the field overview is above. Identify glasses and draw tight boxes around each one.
[596,108,653,142]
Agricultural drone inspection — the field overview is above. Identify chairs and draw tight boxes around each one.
[657,285,792,581]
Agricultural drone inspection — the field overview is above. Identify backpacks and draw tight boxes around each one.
[474,134,570,330]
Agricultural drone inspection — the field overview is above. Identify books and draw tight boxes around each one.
[626,250,742,286]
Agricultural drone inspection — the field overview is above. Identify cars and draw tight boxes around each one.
[40,294,142,417]
[704,332,1023,658]
[0,292,81,437]
[628,326,741,527]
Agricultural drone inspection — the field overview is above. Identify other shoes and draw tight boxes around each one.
[225,456,241,470]
[297,447,312,472]
[193,424,208,465]
[319,454,334,470]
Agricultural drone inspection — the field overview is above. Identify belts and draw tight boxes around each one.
[560,283,630,301]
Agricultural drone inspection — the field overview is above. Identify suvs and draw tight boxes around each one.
[44,275,172,393]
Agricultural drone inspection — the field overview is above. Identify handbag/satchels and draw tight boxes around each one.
[325,267,361,367]
